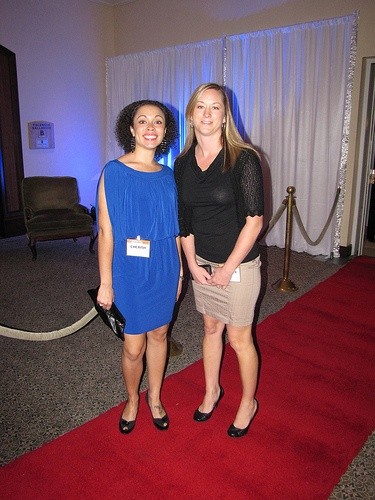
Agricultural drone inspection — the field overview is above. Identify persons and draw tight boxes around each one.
[174,82,265,438]
[96,100,183,434]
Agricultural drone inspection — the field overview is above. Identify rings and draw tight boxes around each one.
[100,304,103,306]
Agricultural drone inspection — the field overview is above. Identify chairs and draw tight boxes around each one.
[21,175,97,261]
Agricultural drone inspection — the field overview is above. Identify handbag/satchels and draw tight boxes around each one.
[87,289,125,341]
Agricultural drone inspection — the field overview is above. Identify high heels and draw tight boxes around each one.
[146,390,169,429]
[227,399,258,437]
[119,396,140,433]
[193,385,224,422]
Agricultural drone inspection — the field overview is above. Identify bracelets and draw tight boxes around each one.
[179,276,184,280]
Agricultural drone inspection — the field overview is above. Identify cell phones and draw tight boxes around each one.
[190,264,212,280]
